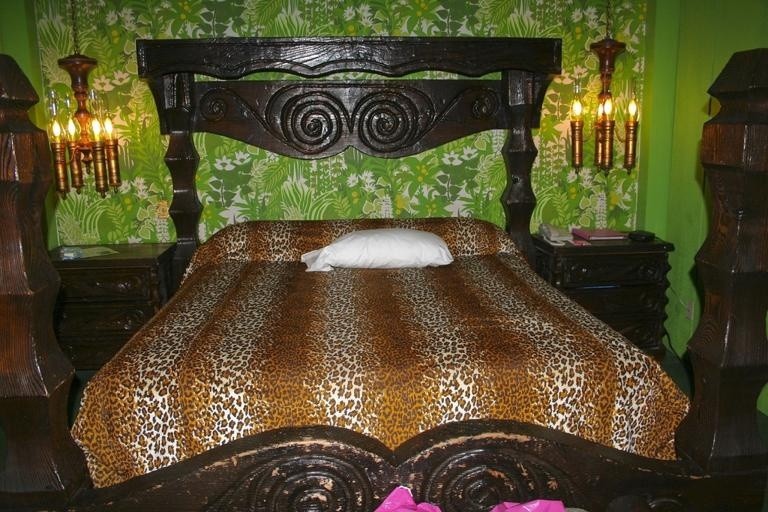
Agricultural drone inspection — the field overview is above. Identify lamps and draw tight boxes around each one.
[569,0,639,178]
[47,1,122,201]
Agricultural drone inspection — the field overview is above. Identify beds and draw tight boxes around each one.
[0,36,768,512]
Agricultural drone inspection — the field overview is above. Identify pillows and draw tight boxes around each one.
[300,227,455,271]
[198,216,520,266]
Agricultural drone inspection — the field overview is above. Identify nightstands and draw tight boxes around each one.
[532,230,675,361]
[42,241,177,428]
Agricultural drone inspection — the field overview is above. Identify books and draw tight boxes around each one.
[572,227,630,241]
[567,238,591,246]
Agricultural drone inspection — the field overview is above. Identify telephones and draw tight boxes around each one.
[539,224,574,241]
